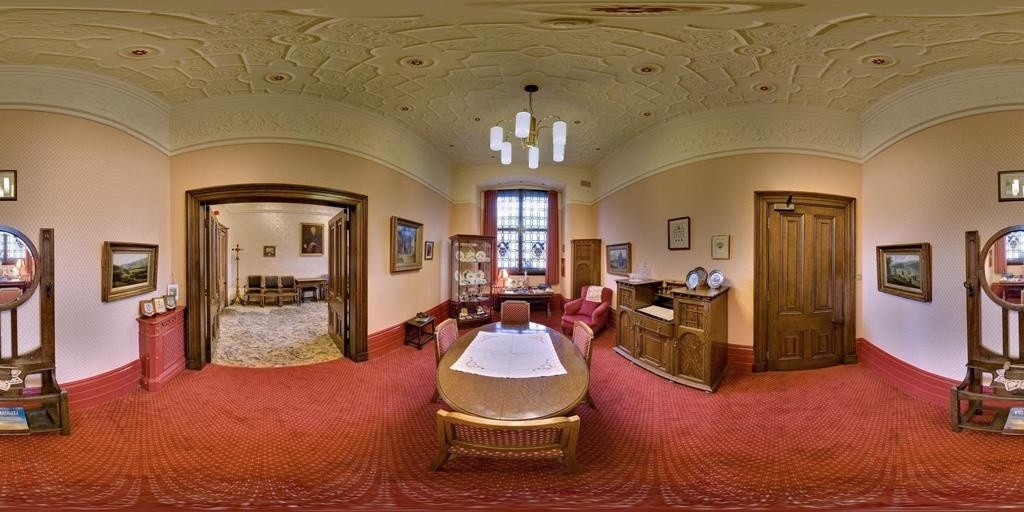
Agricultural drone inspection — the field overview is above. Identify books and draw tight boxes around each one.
[1002,407,1024,434]
[0,406,29,431]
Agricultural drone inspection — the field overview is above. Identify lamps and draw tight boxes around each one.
[499,267,510,291]
[484,80,570,171]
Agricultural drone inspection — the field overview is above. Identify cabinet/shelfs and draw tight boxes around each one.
[612,274,731,395]
[136,305,188,393]
[0,223,72,438]
[950,224,1024,438]
[447,233,496,328]
[570,238,602,300]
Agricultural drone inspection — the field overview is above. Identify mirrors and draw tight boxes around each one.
[1,223,42,312]
[976,224,1023,309]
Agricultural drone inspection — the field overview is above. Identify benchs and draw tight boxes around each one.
[426,408,584,480]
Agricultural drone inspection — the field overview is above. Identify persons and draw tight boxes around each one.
[304,226,321,252]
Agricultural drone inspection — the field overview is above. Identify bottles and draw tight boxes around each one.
[476,285,482,297]
[658,286,671,295]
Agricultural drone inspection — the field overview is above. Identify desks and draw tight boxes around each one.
[294,278,327,304]
[399,311,437,348]
[497,288,554,320]
[434,318,591,425]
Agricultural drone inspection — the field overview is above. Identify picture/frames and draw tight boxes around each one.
[667,215,691,251]
[99,238,160,305]
[995,165,1024,202]
[424,240,435,261]
[872,240,936,306]
[297,219,325,257]
[263,245,276,258]
[605,242,632,277]
[139,283,180,319]
[388,213,425,273]
[710,233,730,261]
[0,169,18,201]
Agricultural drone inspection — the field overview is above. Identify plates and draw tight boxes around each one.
[454,270,485,281]
[455,251,466,262]
[476,250,487,260]
[685,271,700,290]
[694,265,708,286]
[706,269,725,290]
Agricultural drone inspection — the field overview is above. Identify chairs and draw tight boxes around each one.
[497,299,533,325]
[568,318,599,411]
[430,315,459,404]
[243,274,298,308]
[560,283,613,337]
